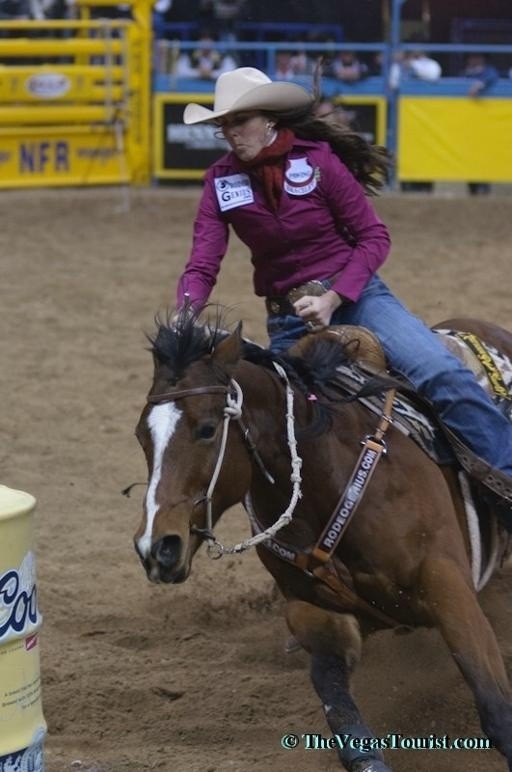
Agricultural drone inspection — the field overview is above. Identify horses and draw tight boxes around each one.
[133,295,512,772]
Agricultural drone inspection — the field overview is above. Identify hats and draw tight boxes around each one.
[179,61,318,132]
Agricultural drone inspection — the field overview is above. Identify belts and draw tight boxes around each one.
[263,268,356,320]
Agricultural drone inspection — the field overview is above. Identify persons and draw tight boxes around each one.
[167,32,377,88]
[461,53,502,200]
[385,36,441,193]
[161,62,511,653]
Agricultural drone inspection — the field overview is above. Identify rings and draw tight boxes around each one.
[308,320,315,330]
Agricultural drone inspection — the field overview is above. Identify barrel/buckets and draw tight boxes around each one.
[0,482,49,760]
